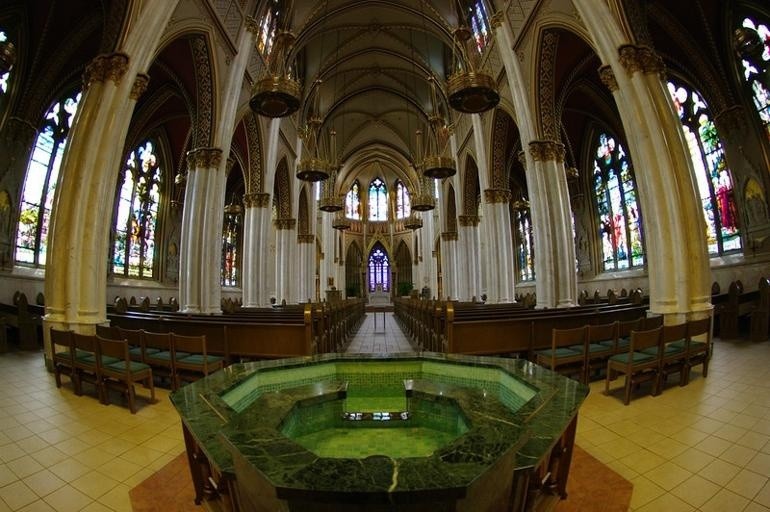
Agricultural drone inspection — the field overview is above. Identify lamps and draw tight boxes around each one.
[560,120,578,179]
[296,0,351,231]
[448,0,500,113]
[247,0,301,119]
[402,0,456,230]
[511,179,530,213]
[223,193,242,215]
[167,127,186,208]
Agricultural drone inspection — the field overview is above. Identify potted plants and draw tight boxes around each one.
[344,283,359,299]
[396,280,412,299]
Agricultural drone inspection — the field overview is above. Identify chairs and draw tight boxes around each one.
[49,324,225,415]
[535,314,712,406]
